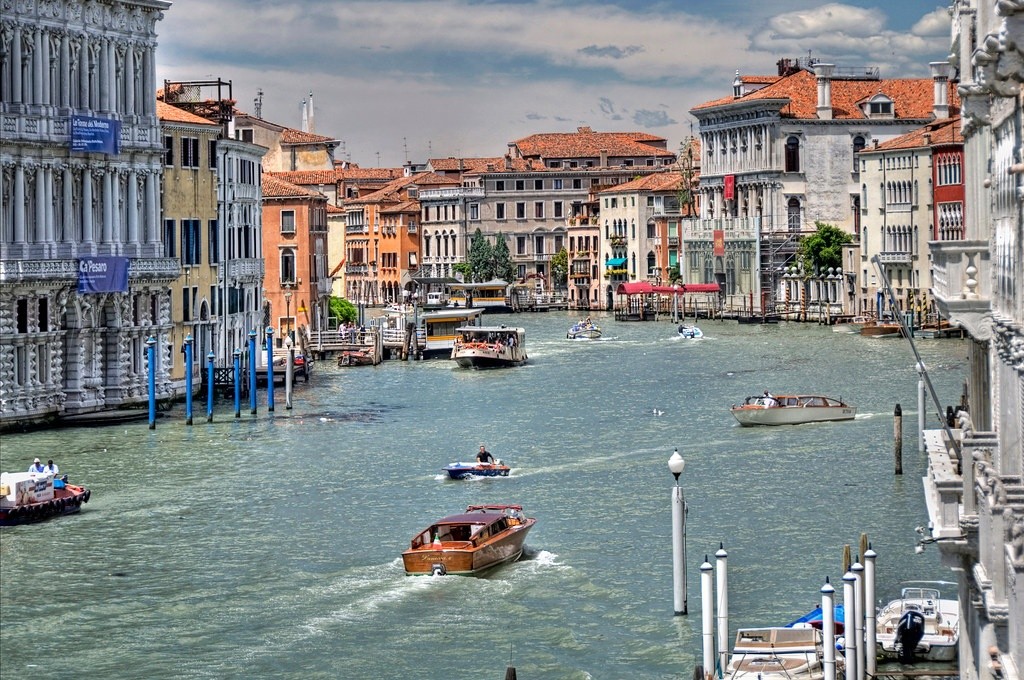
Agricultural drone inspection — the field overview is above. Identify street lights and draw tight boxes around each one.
[284,278,293,336]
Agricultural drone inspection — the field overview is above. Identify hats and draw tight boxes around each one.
[34,458,40,462]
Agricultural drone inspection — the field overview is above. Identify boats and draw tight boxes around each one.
[0,471,90,526]
[566,317,603,340]
[729,389,859,428]
[450,324,529,370]
[859,324,903,337]
[336,347,374,366]
[710,580,958,680]
[441,461,511,480]
[399,502,538,578]
[677,324,704,340]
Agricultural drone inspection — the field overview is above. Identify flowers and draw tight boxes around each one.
[605,269,626,274]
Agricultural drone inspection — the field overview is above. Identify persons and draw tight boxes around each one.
[473,332,515,347]
[359,324,365,344]
[338,321,356,344]
[578,316,591,326]
[763,390,773,405]
[29,458,44,473]
[477,446,494,464]
[678,324,687,338]
[43,460,59,479]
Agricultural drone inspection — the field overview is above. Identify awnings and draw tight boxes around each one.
[605,258,627,266]
